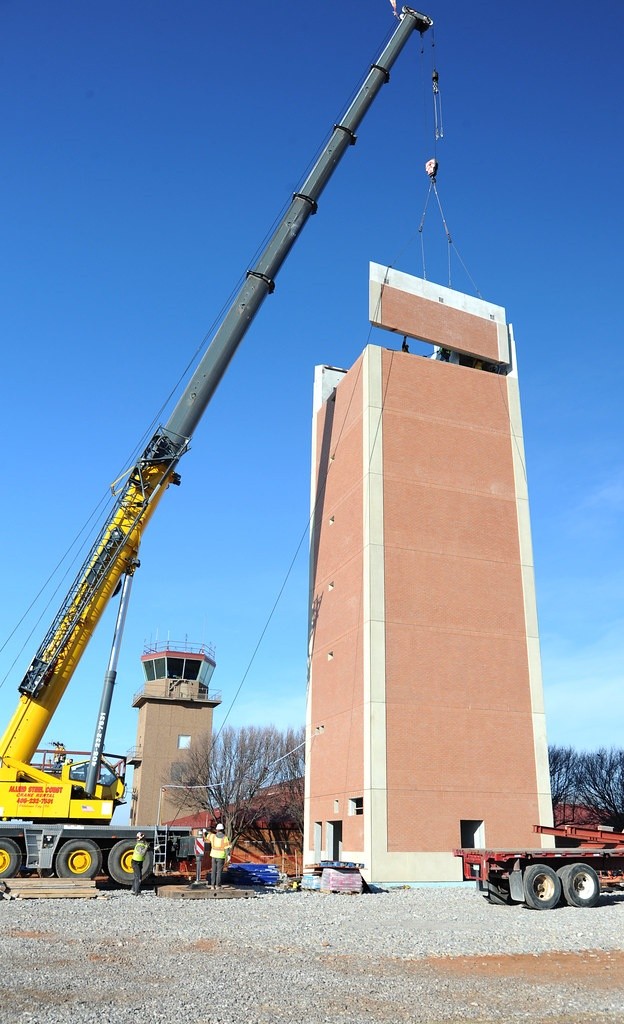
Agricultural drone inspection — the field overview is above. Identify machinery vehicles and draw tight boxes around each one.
[1,0,448,890]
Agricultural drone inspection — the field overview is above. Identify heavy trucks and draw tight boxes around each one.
[452,822,624,909]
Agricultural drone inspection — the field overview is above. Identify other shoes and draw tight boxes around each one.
[130,890,140,895]
[216,885,224,890]
[211,886,216,890]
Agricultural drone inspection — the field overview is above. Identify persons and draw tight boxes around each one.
[131,832,150,898]
[48,740,76,774]
[436,346,452,363]
[202,823,231,889]
[473,359,508,374]
[401,335,410,354]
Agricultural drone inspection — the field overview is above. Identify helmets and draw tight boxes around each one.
[137,832,145,841]
[216,823,224,830]
